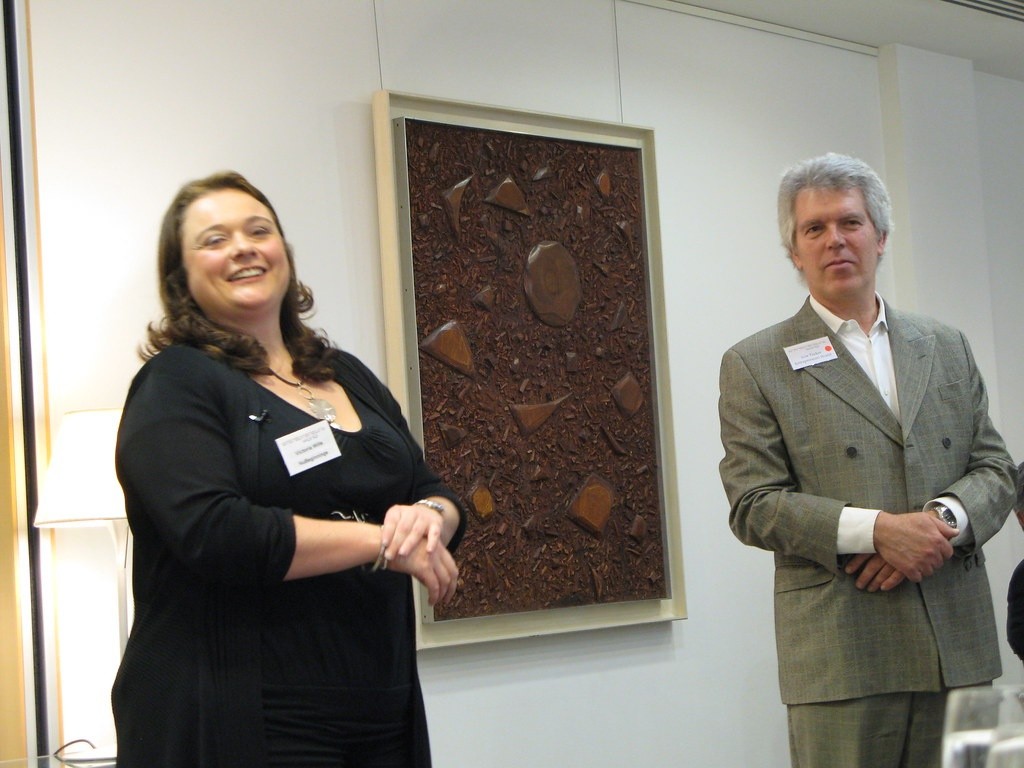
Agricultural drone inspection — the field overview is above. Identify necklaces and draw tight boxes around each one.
[269,345,337,422]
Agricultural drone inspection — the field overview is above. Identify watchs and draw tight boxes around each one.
[930,505,958,540]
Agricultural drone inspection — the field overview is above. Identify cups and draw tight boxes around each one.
[939,684,1024,768]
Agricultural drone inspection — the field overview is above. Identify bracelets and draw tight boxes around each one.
[416,498,446,513]
[362,525,390,573]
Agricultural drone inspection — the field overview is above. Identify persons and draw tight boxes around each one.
[1006,461,1024,662]
[718,153,1018,768]
[112,170,468,768]
